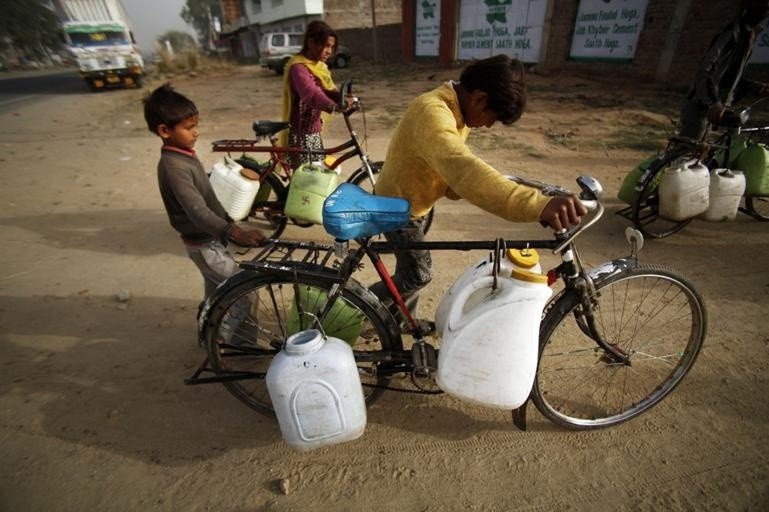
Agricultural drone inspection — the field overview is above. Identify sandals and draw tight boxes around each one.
[403,320,435,336]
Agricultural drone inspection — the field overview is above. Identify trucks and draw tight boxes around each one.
[63,20,144,92]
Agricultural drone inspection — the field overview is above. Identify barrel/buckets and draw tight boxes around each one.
[283,282,365,351]
[208,158,261,223]
[435,248,542,339]
[658,156,712,219]
[239,155,281,199]
[738,142,769,196]
[435,267,553,410]
[304,157,341,175]
[265,330,367,452]
[715,134,751,166]
[284,162,337,228]
[618,154,663,203]
[704,168,746,223]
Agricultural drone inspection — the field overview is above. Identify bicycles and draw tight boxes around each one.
[183,176,708,431]
[616,105,769,238]
[208,80,433,247]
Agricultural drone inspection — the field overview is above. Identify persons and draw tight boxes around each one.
[675,0,769,172]
[264,19,354,229]
[343,54,588,341]
[144,88,266,354]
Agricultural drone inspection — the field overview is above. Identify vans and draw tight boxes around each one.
[259,32,351,73]
